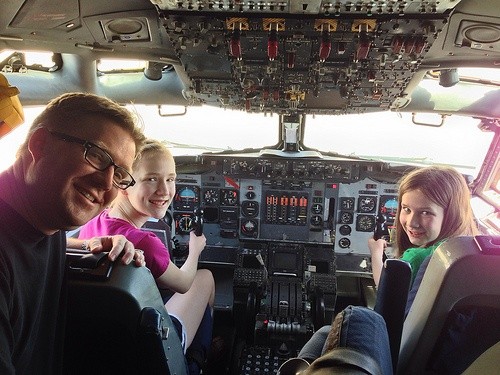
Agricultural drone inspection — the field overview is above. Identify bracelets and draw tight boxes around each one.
[81,240,90,250]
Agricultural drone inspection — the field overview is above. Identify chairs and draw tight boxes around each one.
[66,248,214,375]
[362,235,500,375]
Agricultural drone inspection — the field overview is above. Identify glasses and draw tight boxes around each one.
[43,126,138,192]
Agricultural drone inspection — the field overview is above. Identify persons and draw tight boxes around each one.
[0,92,146,375]
[78,138,216,355]
[367,165,473,293]
